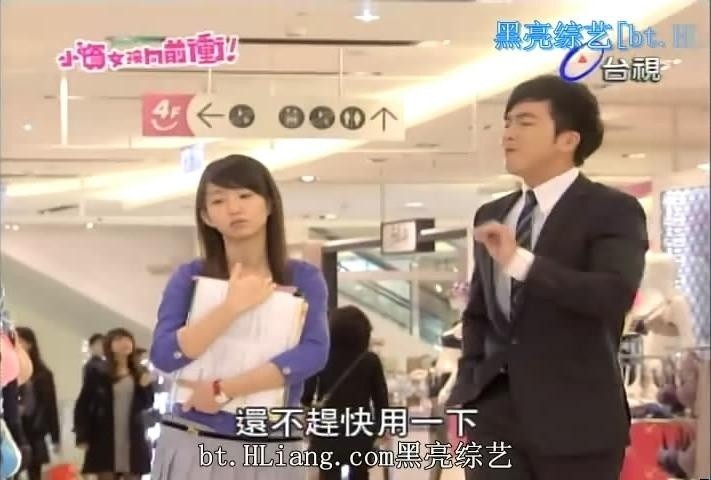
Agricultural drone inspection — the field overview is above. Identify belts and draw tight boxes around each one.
[162,421,303,442]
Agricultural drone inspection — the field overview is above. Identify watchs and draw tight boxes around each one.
[294,304,392,480]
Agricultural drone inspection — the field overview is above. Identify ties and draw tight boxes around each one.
[510,191,538,319]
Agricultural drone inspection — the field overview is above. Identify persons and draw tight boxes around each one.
[147,153,331,480]
[133,347,148,368]
[9,326,62,479]
[73,326,156,479]
[436,72,652,479]
[68,333,111,452]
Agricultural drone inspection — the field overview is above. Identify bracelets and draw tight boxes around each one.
[212,380,230,405]
